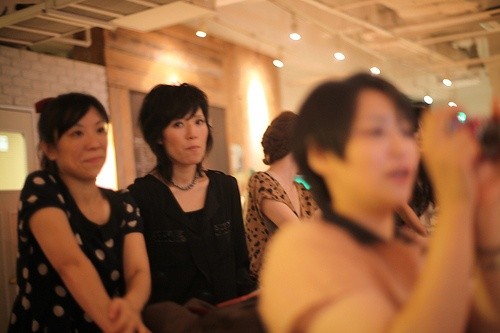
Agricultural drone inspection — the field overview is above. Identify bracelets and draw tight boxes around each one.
[476,244,500,272]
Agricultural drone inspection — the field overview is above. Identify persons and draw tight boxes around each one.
[408,98,439,220]
[256,71,500,333]
[245,110,320,288]
[126,83,252,315]
[7,92,154,333]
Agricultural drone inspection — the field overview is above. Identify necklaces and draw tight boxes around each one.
[169,171,199,191]
